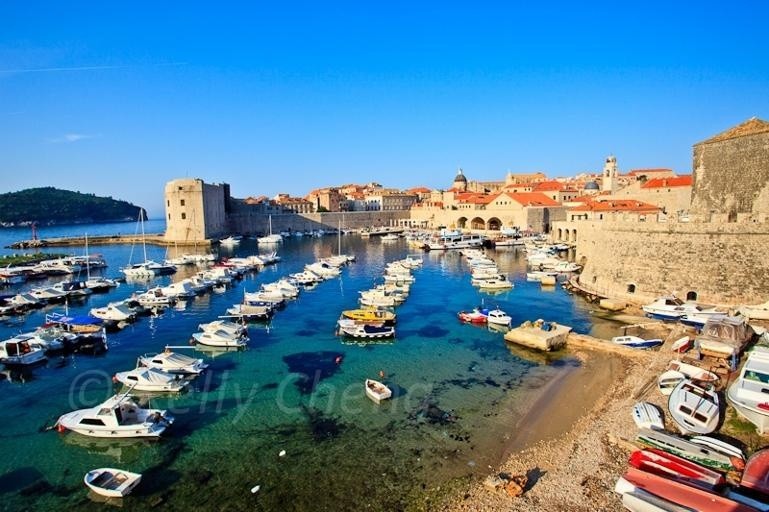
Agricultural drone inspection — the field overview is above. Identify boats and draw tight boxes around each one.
[613,297,769,512]
[0,207,581,497]
